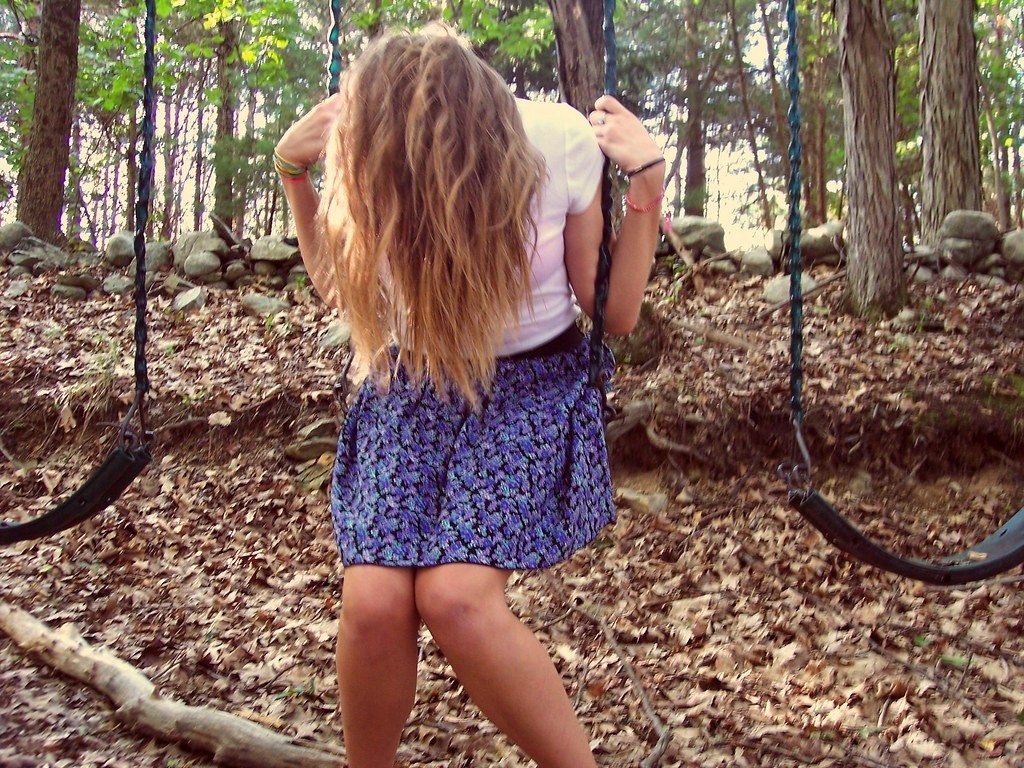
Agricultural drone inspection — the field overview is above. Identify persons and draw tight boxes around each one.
[270,22,665,768]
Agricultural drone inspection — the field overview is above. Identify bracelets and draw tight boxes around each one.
[624,158,664,186]
[273,147,310,183]
[624,186,664,211]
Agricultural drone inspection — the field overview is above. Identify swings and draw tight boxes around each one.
[0,0,158,547]
[328,1,619,420]
[775,0,1023,588]
